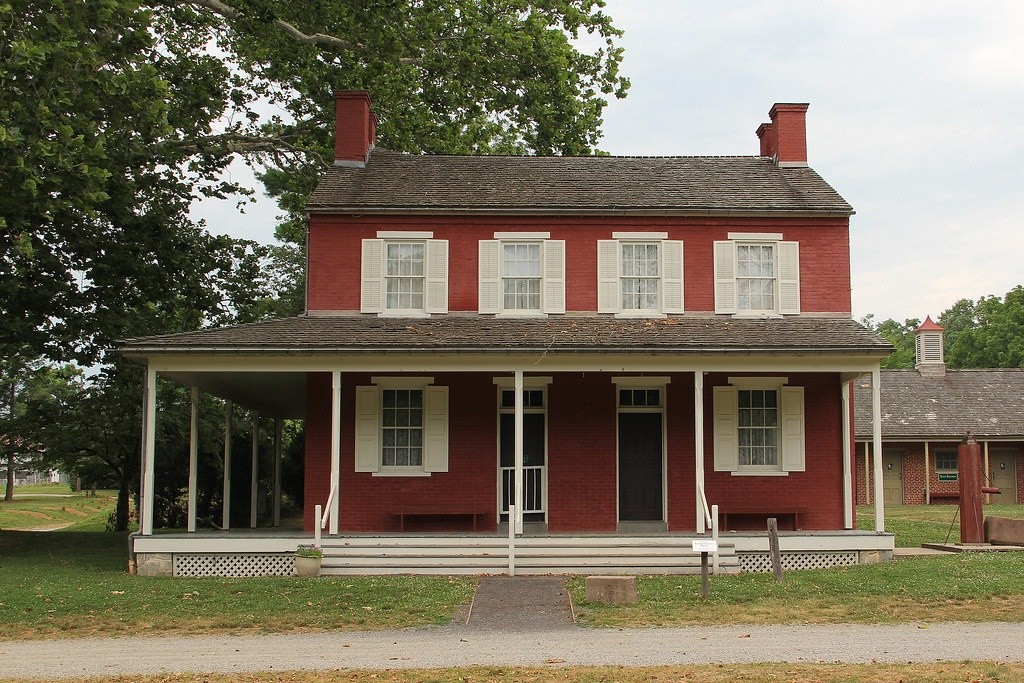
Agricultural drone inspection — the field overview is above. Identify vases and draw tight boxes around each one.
[293,553,323,578]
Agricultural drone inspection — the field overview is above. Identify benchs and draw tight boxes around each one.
[931,494,960,504]
[718,506,808,532]
[390,507,489,532]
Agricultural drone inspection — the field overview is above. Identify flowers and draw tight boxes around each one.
[295,544,323,558]
